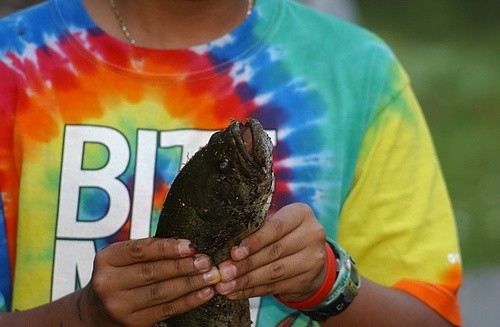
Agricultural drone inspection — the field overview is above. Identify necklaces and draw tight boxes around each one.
[108,0,253,48]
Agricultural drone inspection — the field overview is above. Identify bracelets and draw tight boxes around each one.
[274,240,337,311]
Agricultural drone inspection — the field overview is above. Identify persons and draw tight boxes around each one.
[0,0,464,326]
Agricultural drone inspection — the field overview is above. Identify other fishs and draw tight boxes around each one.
[154,117,276,327]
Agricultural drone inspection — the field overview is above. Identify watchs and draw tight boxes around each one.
[301,237,361,322]
